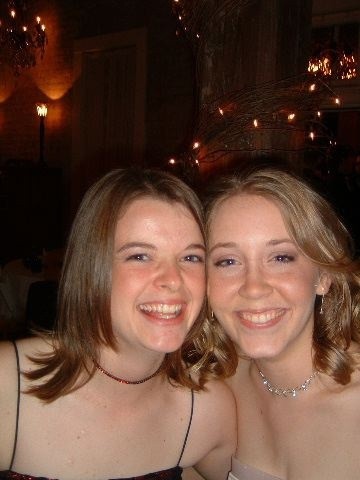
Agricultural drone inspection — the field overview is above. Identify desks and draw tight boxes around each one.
[0,259,58,330]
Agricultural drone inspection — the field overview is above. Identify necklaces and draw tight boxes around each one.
[247,353,324,396]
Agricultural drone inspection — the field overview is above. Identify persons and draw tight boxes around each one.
[0,160,236,478]
[185,167,360,480]
